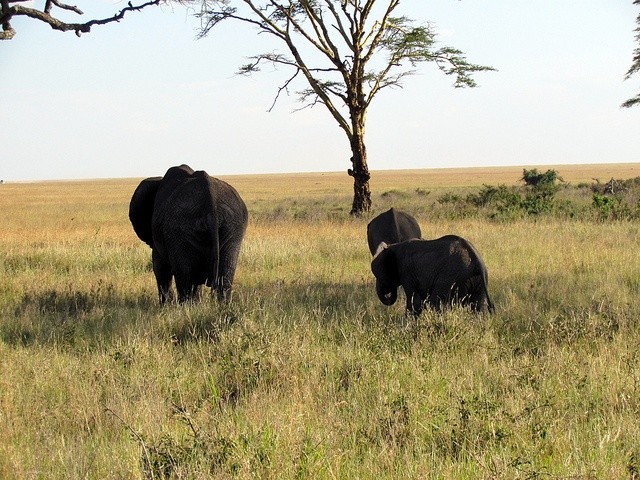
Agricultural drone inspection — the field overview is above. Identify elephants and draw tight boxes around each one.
[371,234,495,319]
[366,207,421,256]
[128,164,249,306]
